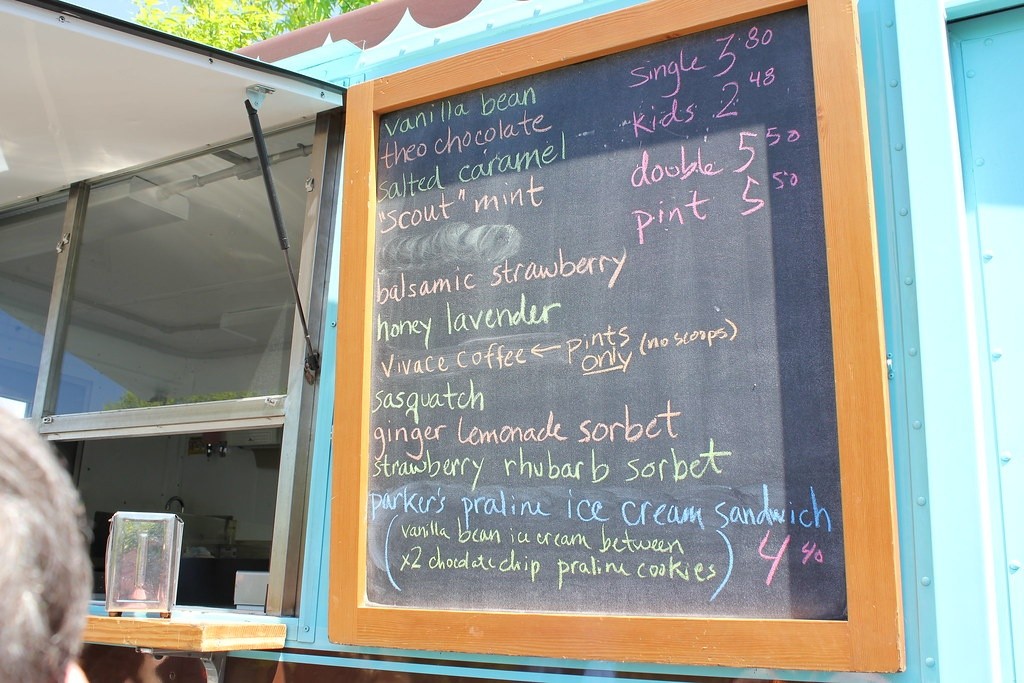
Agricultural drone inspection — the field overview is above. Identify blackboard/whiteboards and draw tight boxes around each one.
[327,0,907,673]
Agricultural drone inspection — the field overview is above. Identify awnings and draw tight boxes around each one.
[0,0,347,358]
[0,112,348,402]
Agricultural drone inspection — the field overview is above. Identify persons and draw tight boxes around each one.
[1,406,92,682]
